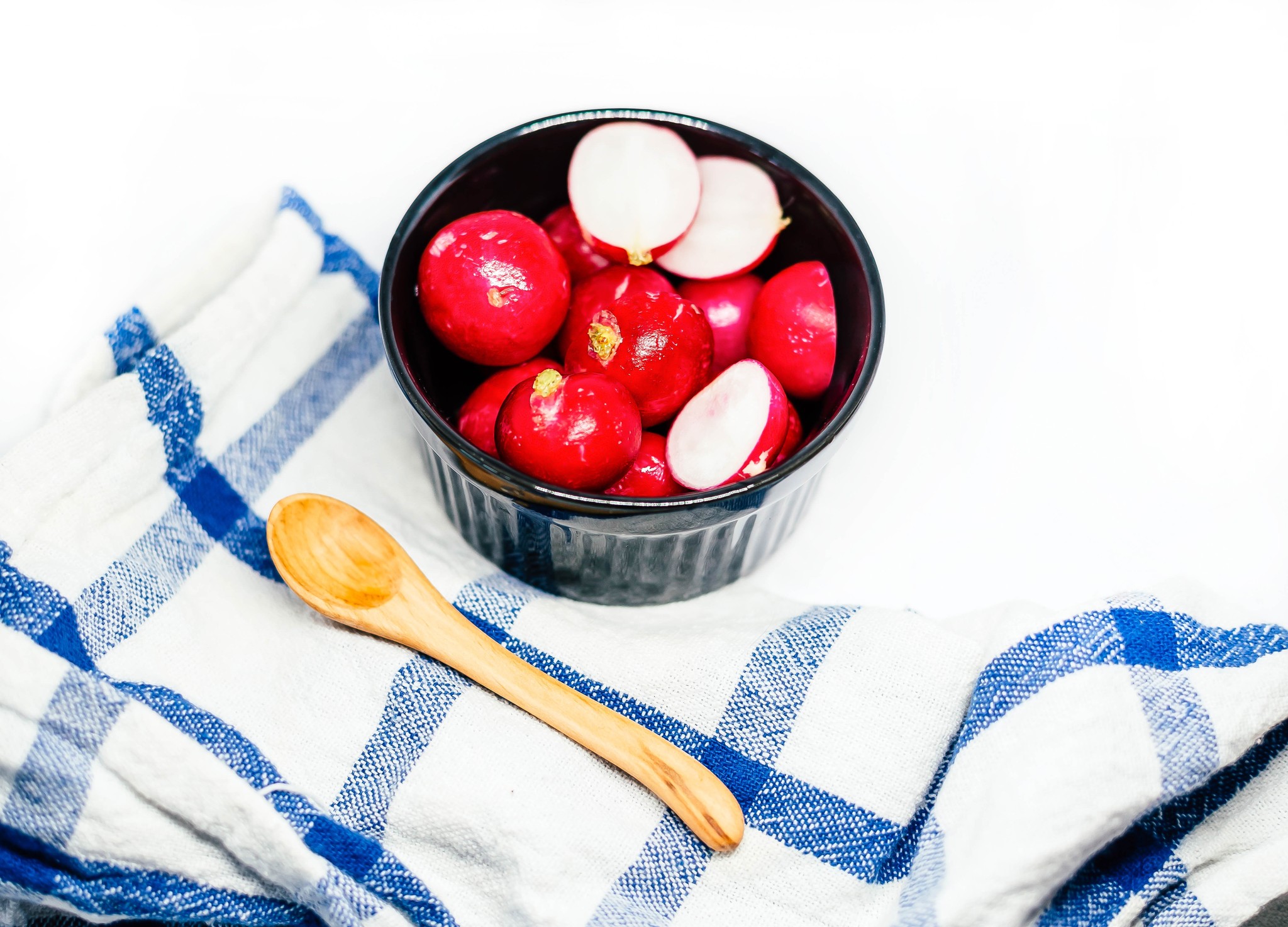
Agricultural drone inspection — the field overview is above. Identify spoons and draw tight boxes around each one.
[265,492,747,853]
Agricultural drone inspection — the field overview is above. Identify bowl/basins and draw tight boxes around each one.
[378,106,887,609]
